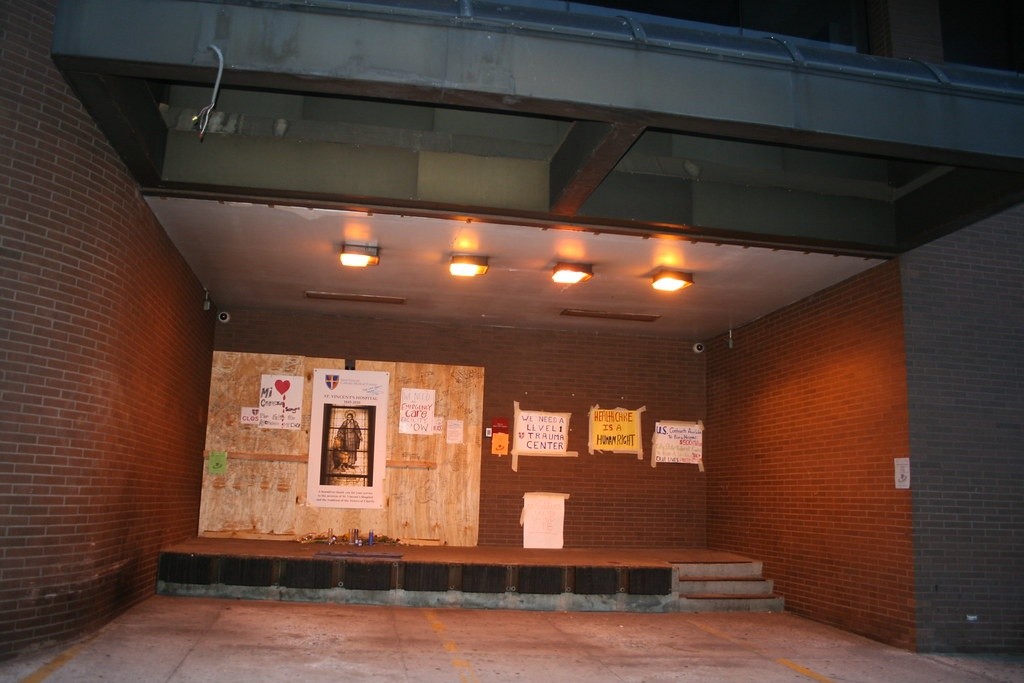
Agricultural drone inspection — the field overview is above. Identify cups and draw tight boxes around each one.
[369,530,374,545]
[358,539,362,546]
[349,529,354,544]
[328,528,333,543]
[354,529,359,545]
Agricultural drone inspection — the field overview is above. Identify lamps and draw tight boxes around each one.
[203,291,210,310]
[729,330,735,348]
[340,245,380,269]
[450,256,488,275]
[552,262,593,284]
[652,270,694,290]
